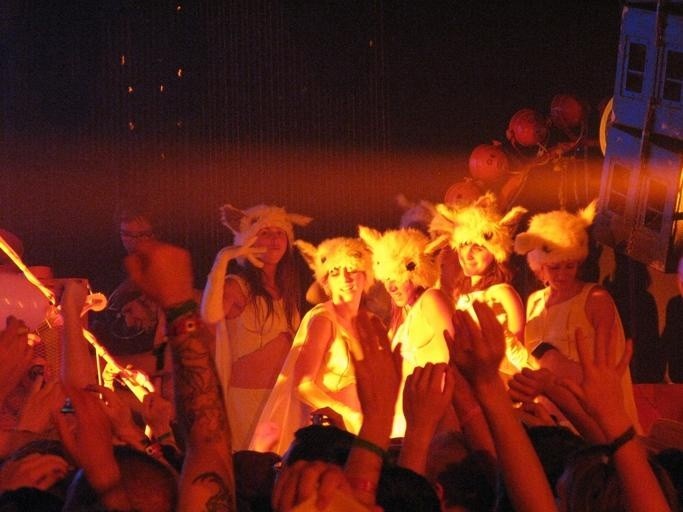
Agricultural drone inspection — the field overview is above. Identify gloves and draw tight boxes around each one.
[201,237,267,324]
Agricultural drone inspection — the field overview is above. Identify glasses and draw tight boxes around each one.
[115,228,155,241]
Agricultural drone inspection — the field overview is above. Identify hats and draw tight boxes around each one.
[400,192,529,265]
[294,237,374,292]
[358,225,450,290]
[108,279,143,313]
[220,204,314,259]
[514,198,597,284]
